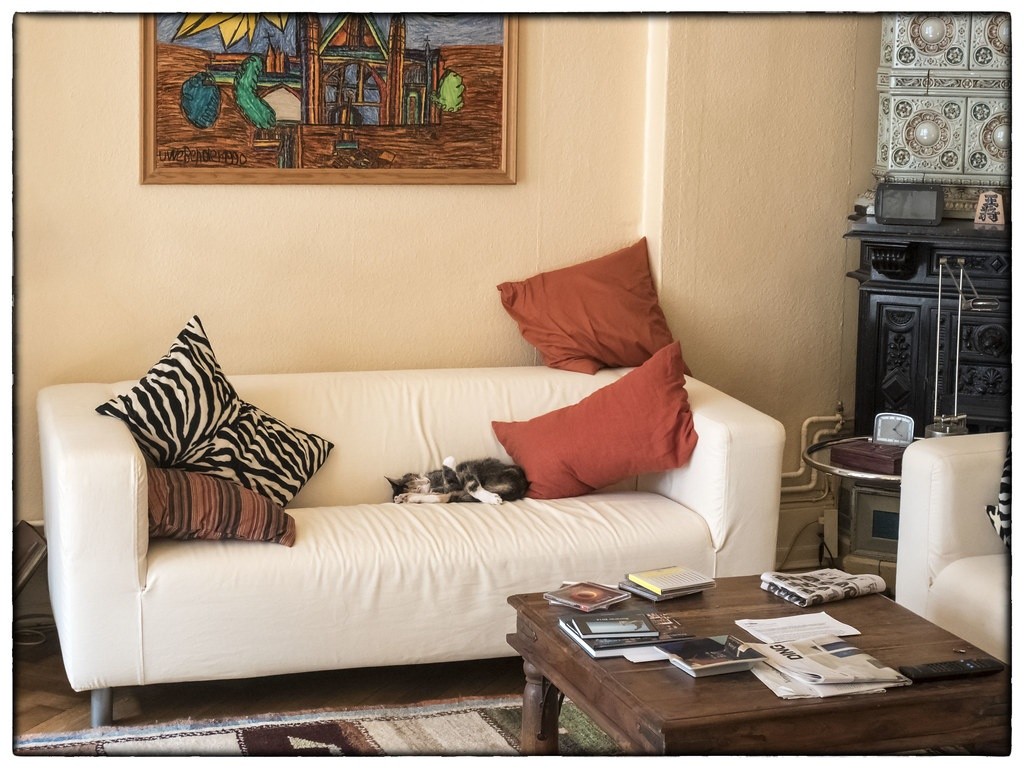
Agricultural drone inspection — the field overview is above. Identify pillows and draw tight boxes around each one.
[147,468,294,548]
[984,436,1009,550]
[492,340,697,500]
[495,237,694,376]
[96,314,335,509]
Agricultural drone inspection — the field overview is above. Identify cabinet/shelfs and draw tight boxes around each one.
[849,214,1012,493]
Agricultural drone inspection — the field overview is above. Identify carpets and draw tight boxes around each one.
[13,691,974,757]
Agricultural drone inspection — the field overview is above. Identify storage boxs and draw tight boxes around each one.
[831,440,906,475]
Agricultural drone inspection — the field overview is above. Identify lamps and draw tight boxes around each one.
[924,258,1001,438]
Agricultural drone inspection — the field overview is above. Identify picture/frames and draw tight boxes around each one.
[139,12,519,185]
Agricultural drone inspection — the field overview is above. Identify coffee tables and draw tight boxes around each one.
[506,570,1010,756]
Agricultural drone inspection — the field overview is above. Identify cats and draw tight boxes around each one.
[384,456,533,506]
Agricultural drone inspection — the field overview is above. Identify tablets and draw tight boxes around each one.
[875,183,943,226]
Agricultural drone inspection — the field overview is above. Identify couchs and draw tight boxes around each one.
[35,365,784,730]
[894,432,1009,667]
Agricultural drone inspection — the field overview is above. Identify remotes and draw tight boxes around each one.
[899,659,1005,682]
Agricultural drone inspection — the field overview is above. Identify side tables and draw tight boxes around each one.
[801,436,925,483]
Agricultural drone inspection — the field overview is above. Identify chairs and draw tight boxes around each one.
[13,520,47,602]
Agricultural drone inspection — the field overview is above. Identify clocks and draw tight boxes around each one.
[873,413,914,448]
